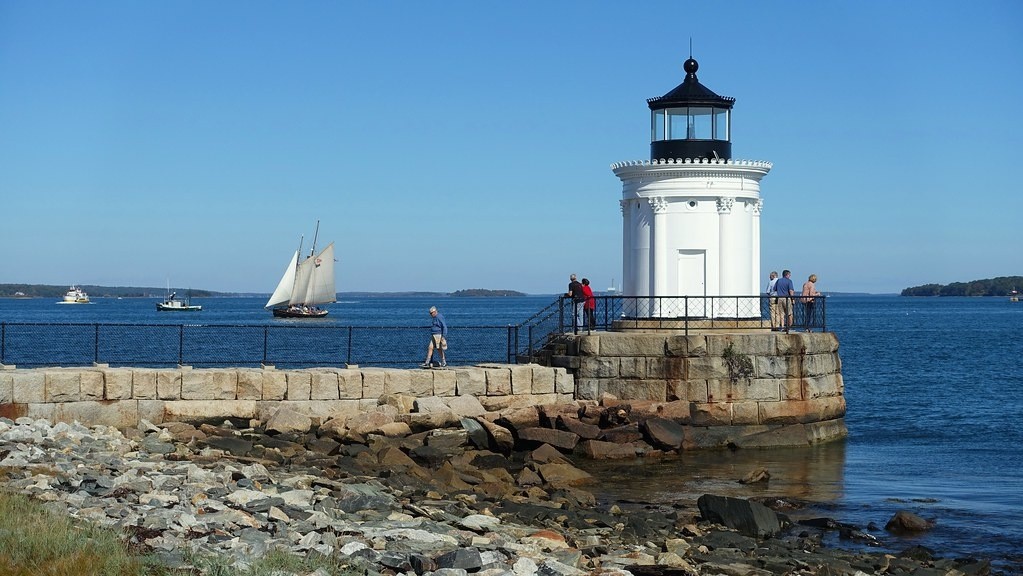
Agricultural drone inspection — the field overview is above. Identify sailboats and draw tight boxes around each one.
[606,277,616,292]
[261,219,340,318]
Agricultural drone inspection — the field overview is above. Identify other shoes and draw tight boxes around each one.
[585,329,596,332]
[771,328,781,332]
[573,329,583,333]
[782,328,790,332]
[805,328,814,333]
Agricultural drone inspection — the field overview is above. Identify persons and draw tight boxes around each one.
[801,274,821,333]
[564,275,585,333]
[419,305,449,367]
[767,271,781,331]
[772,270,797,333]
[581,278,597,334]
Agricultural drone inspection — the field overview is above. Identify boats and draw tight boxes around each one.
[154,276,202,313]
[59,284,92,304]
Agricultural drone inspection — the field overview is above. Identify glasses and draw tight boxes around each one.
[430,312,433,314]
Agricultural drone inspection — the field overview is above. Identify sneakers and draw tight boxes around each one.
[441,362,447,366]
[418,363,430,368]
